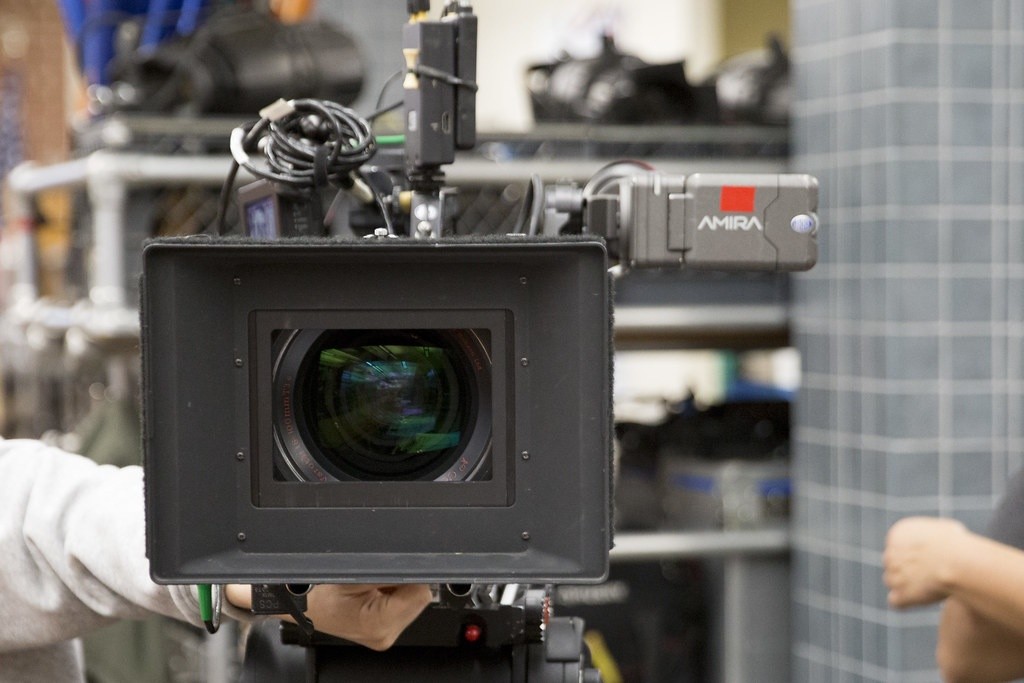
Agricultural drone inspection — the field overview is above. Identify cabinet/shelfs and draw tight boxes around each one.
[8,131,792,683]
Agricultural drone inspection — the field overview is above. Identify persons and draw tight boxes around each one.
[881,463,1024,683]
[0,435,430,682]
